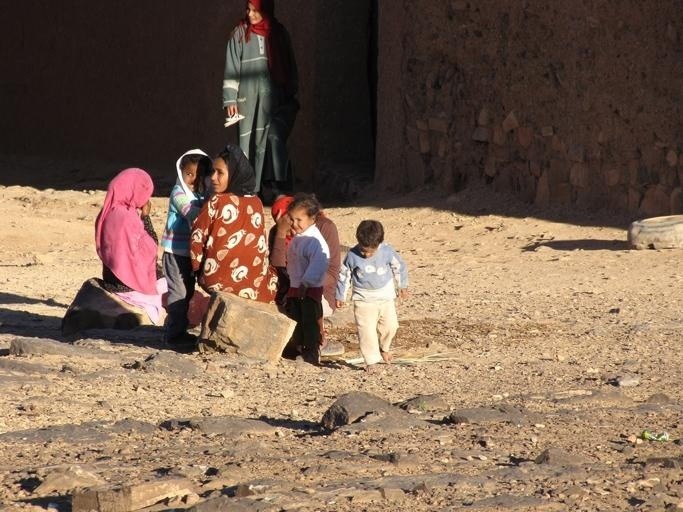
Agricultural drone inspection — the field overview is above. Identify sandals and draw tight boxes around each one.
[223,112,245,128]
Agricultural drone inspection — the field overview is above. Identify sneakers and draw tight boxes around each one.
[160,329,198,348]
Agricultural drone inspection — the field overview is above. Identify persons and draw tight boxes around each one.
[185,142,279,327]
[265,192,340,321]
[278,195,330,363]
[94,167,169,327]
[161,147,216,348]
[221,0,300,206]
[335,220,409,376]
